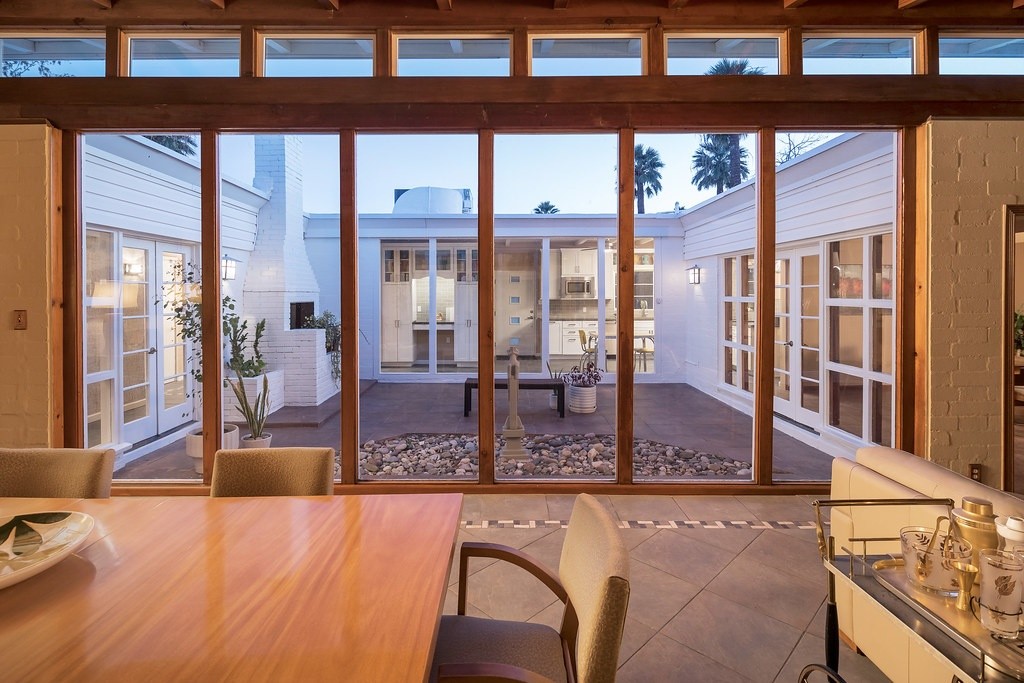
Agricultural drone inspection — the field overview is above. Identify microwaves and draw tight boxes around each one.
[560,277,595,298]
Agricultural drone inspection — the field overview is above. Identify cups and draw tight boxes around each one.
[951,561,979,612]
[898,525,973,597]
[978,545,1024,640]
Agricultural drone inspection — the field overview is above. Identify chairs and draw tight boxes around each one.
[633,348,654,372]
[209,446,335,497]
[0,447,116,499]
[428,493,631,683]
[579,330,609,373]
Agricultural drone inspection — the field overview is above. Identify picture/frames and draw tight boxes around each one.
[832,263,895,316]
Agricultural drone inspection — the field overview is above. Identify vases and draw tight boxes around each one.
[568,383,597,414]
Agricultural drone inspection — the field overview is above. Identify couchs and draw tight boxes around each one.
[829,446,1024,683]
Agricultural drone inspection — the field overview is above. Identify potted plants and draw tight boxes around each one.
[546,360,564,410]
[223,316,285,422]
[226,368,273,449]
[151,259,239,474]
[300,308,341,390]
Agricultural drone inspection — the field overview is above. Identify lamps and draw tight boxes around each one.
[685,264,700,285]
[124,263,144,276]
[221,254,243,281]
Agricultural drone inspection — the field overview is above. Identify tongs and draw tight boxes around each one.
[915,514,953,580]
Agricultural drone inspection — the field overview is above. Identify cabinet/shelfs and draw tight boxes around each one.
[381,243,411,284]
[453,284,479,367]
[382,284,418,368]
[538,248,615,300]
[583,321,598,360]
[549,321,563,360]
[454,243,478,285]
[563,321,583,360]
[410,242,454,279]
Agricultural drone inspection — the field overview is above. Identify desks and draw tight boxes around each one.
[806,498,1024,683]
[588,330,654,372]
[0,492,464,683]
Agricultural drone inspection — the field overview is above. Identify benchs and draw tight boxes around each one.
[463,378,565,419]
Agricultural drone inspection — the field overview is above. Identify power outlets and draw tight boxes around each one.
[418,306,422,313]
[968,464,982,483]
[446,337,450,343]
[583,306,586,312]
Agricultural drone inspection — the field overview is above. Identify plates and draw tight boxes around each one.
[0,511,95,590]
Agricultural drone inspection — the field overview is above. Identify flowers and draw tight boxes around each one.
[563,362,604,387]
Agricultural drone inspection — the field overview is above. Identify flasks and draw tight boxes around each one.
[951,496,999,586]
